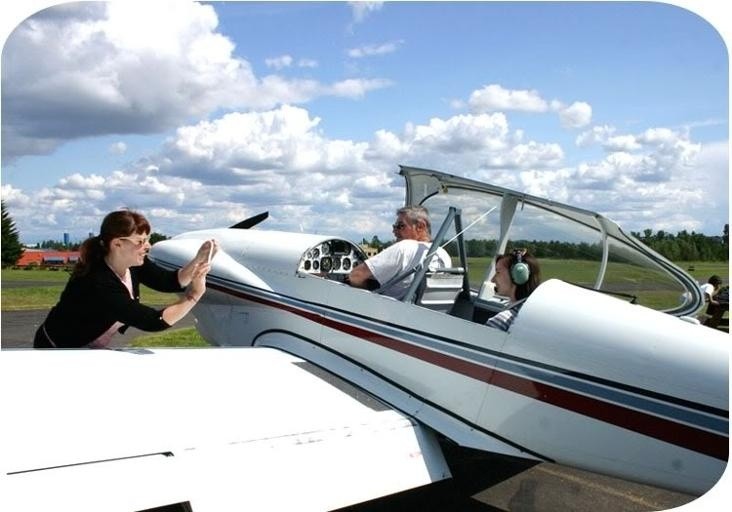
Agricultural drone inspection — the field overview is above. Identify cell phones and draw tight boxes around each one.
[206,240,214,265]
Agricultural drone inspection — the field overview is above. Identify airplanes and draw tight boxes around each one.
[1,163,728,512]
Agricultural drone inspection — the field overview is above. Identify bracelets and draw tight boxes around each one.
[186,293,197,303]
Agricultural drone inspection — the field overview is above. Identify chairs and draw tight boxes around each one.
[392,263,466,319]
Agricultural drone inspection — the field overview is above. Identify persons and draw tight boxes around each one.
[484,247,543,331]
[34,210,219,348]
[680,276,723,318]
[349,205,453,299]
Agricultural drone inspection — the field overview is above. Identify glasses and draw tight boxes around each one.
[119,236,150,245]
[393,224,406,229]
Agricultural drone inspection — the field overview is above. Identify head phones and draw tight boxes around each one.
[510,249,530,285]
